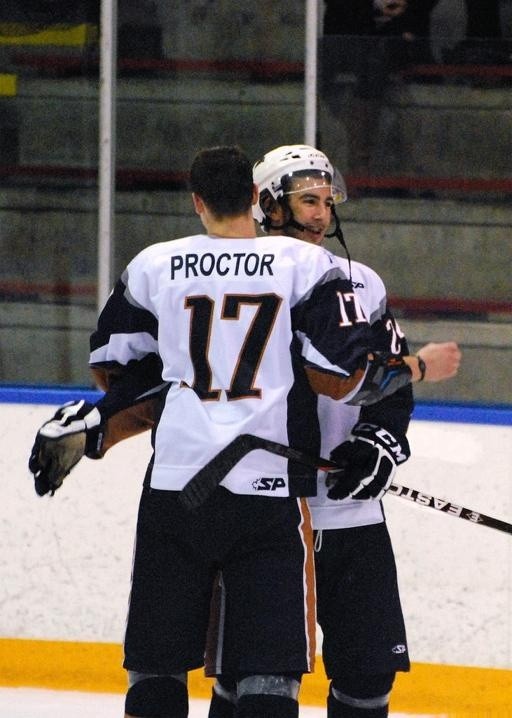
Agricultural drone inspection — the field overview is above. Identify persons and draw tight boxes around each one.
[33,143,413,716]
[88,147,461,718]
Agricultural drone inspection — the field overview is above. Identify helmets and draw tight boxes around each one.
[252,144,334,233]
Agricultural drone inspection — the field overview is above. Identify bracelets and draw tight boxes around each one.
[414,354,427,383]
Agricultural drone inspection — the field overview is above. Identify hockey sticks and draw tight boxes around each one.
[179,432,510,533]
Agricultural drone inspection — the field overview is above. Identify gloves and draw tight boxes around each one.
[29,399,105,496]
[325,420,410,503]
